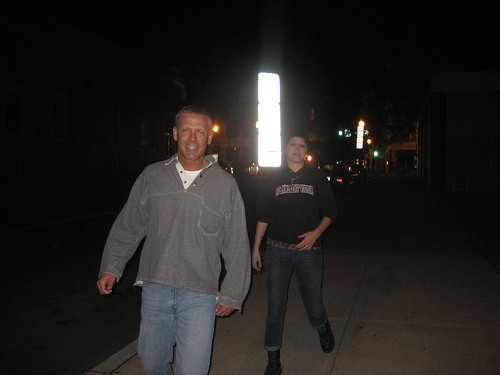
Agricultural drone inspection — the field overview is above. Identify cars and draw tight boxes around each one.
[327,169,361,200]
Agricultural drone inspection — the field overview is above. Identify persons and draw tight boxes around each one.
[97,105,251,375]
[251,130,338,375]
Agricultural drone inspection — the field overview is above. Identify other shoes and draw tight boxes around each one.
[264,362,282,375]
[317,319,336,353]
